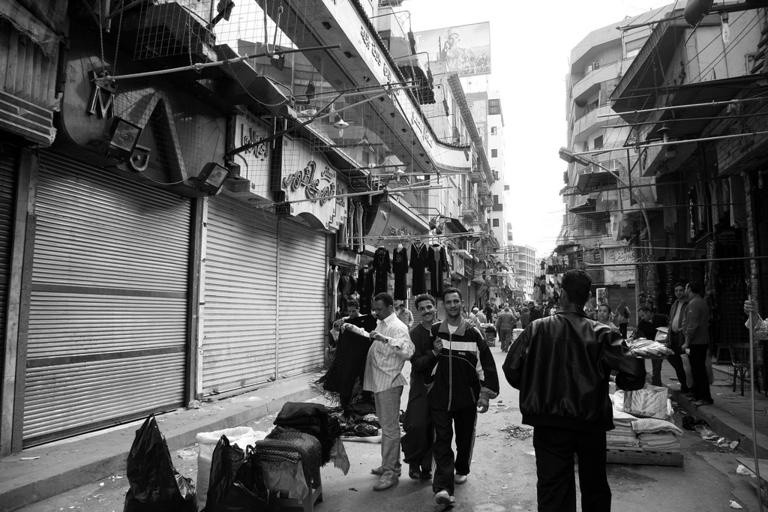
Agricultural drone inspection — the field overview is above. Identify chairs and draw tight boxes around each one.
[729,344,762,396]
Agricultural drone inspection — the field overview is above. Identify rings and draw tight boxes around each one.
[438,344,442,347]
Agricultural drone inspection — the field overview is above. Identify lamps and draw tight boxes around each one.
[106,115,143,156]
[195,160,231,195]
[419,213,445,235]
[409,206,441,230]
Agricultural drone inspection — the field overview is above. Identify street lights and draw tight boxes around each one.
[558,146,657,325]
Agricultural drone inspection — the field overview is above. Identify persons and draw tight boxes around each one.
[399,294,443,481]
[668,282,693,397]
[463,276,667,352]
[424,287,499,508]
[680,281,714,406]
[744,299,767,340]
[502,268,646,512]
[341,293,415,492]
[396,303,414,331]
[628,306,691,393]
[331,299,366,423]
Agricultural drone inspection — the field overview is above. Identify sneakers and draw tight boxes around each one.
[422,464,432,479]
[435,490,455,505]
[373,470,399,490]
[409,464,421,480]
[455,474,467,483]
[372,465,401,476]
